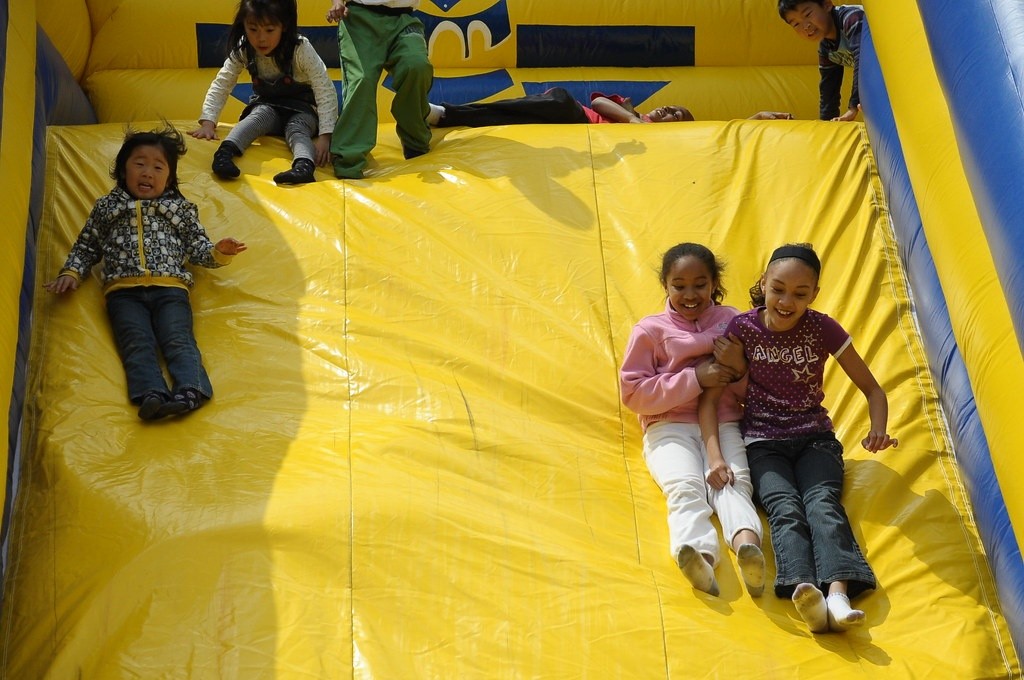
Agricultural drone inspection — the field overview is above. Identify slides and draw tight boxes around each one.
[2,0,1024,679]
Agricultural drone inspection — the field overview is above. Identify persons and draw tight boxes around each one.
[426,87,794,128]
[620,242,764,597]
[699,242,898,633]
[777,0,863,121]
[42,118,248,422]
[186,0,338,183]
[326,0,433,179]
